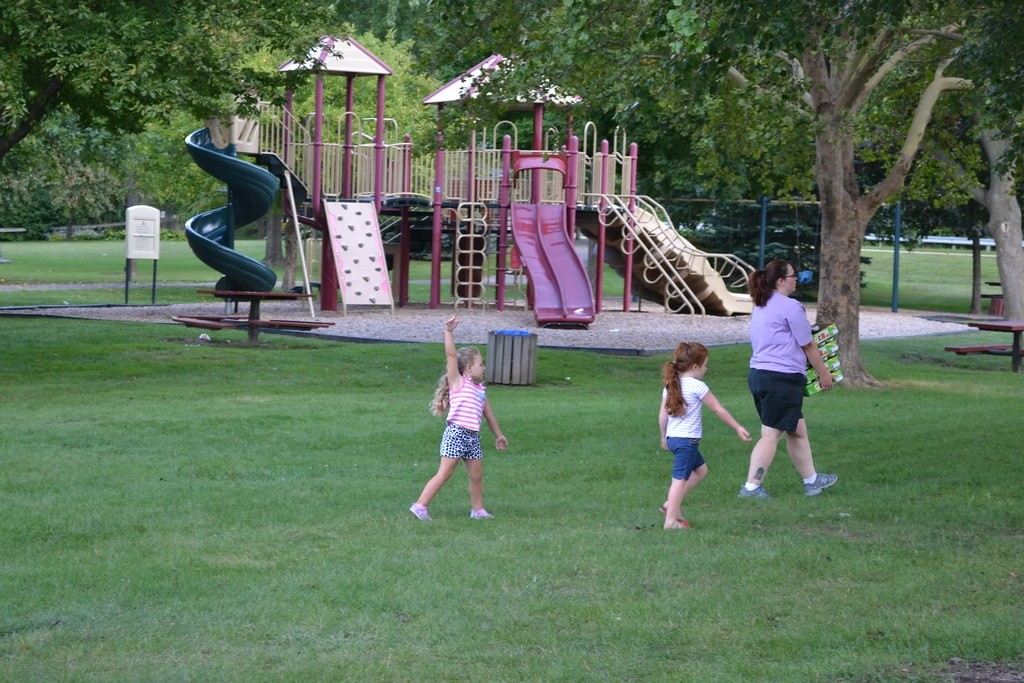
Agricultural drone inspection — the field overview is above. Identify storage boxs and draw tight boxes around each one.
[803,321,844,397]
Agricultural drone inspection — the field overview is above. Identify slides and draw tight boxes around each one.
[507,201,598,325]
[183,126,281,303]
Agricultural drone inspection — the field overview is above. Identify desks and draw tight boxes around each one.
[197,290,316,345]
[969,322,1024,373]
[985,281,1002,285]
[0,227,28,264]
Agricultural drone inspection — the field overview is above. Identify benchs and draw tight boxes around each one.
[944,344,1013,355]
[171,313,335,331]
[980,294,1004,315]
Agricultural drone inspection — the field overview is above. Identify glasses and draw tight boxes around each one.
[783,273,797,277]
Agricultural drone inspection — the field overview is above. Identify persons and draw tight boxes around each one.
[737,258,839,500]
[656,341,754,532]
[408,315,509,522]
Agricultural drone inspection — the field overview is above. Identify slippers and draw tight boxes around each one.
[658,507,682,520]
[664,521,692,529]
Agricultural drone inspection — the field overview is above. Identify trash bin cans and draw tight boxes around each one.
[486,329,538,385]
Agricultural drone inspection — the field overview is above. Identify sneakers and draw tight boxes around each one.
[803,473,839,497]
[471,508,494,519]
[410,503,432,520]
[738,484,772,500]
